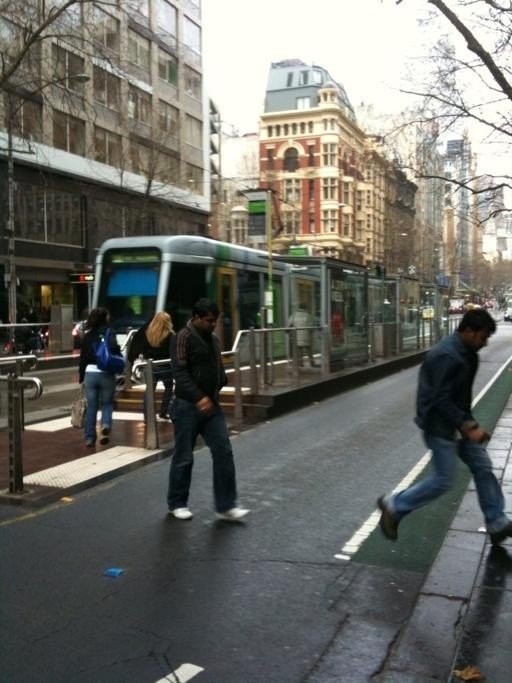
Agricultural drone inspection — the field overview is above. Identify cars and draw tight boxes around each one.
[1,322,47,371]
[36,316,92,351]
[418,281,512,321]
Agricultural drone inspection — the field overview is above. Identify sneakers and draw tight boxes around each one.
[167,507,194,521]
[159,412,169,419]
[377,494,398,542]
[489,520,512,545]
[100,426,110,445]
[214,507,252,520]
[85,438,95,446]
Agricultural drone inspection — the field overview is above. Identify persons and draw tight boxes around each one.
[375,307,512,545]
[128,311,177,423]
[77,306,123,447]
[166,297,252,521]
[286,301,321,368]
[330,300,345,369]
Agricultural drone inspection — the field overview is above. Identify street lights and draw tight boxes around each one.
[3,72,91,362]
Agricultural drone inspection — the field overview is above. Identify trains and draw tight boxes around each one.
[67,233,361,388]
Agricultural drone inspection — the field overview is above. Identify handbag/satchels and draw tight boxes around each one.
[71,397,86,428]
[95,340,129,375]
